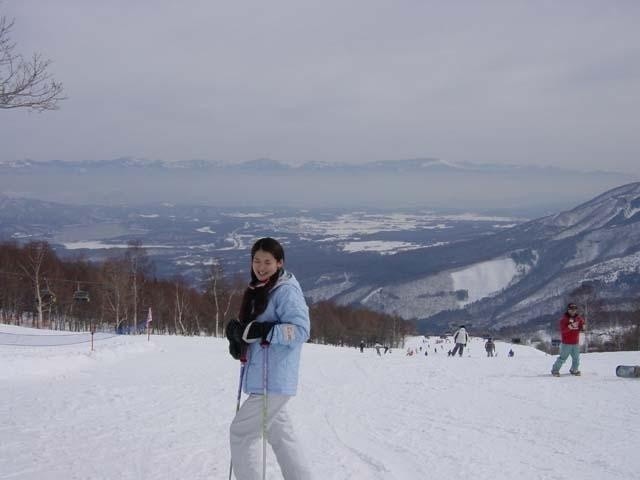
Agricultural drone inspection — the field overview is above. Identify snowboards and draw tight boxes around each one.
[616,366,639,377]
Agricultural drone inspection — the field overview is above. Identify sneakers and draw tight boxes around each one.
[551,370,560,377]
[569,369,580,374]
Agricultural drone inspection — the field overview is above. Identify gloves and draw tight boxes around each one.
[246,321,275,345]
[226,319,244,359]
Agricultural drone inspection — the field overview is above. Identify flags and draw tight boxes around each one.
[145,306,153,324]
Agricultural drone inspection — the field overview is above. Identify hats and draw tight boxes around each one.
[567,303,577,310]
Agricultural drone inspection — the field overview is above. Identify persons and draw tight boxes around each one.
[509,349,514,357]
[451,325,469,357]
[484,337,496,357]
[383,346,388,354]
[374,342,383,356]
[359,340,365,353]
[224,237,316,480]
[549,302,588,378]
